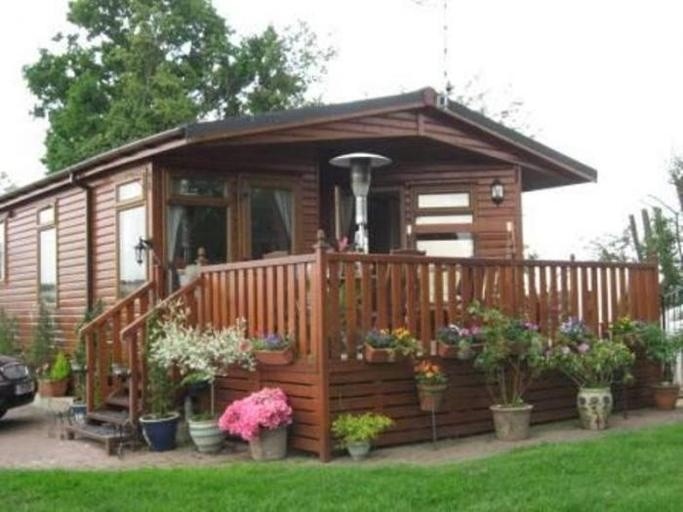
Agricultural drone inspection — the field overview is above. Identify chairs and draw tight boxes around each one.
[357,248,426,329]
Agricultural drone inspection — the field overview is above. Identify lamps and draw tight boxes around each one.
[133,236,151,264]
[489,177,505,209]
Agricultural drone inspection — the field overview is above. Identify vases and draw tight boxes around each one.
[363,344,401,364]
[414,382,448,412]
[247,424,288,462]
[252,343,297,366]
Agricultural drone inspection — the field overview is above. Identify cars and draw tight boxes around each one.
[0,354,39,418]
[660,304,683,344]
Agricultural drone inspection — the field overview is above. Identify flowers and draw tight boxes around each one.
[365,326,427,358]
[413,360,448,384]
[217,388,293,442]
[236,331,292,353]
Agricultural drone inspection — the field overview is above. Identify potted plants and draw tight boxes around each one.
[330,411,394,459]
[435,297,682,442]
[36,297,256,454]
